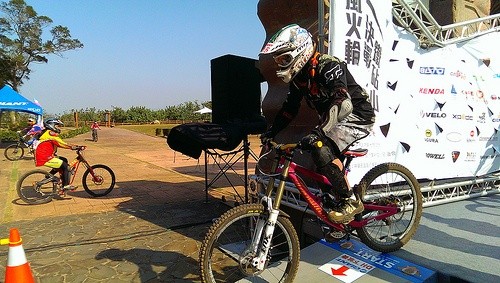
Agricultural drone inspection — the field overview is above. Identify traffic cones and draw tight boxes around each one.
[4,226,36,283]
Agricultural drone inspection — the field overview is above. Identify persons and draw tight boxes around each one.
[17,118,42,153]
[90,121,102,138]
[34,118,82,190]
[260,24,374,223]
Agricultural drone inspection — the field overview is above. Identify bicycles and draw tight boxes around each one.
[4,132,35,161]
[16,145,115,205]
[92,128,100,142]
[197,137,423,283]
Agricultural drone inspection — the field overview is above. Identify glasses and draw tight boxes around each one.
[273,36,311,67]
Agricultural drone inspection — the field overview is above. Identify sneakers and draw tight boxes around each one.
[326,231,339,243]
[63,185,78,190]
[334,194,363,223]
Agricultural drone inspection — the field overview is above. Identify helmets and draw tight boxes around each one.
[44,117,63,134]
[260,24,316,83]
[28,118,36,126]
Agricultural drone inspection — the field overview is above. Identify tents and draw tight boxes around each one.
[0,85,44,127]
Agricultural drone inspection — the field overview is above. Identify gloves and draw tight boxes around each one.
[261,126,279,146]
[294,130,328,152]
[73,145,81,150]
[17,131,22,134]
[22,135,27,139]
